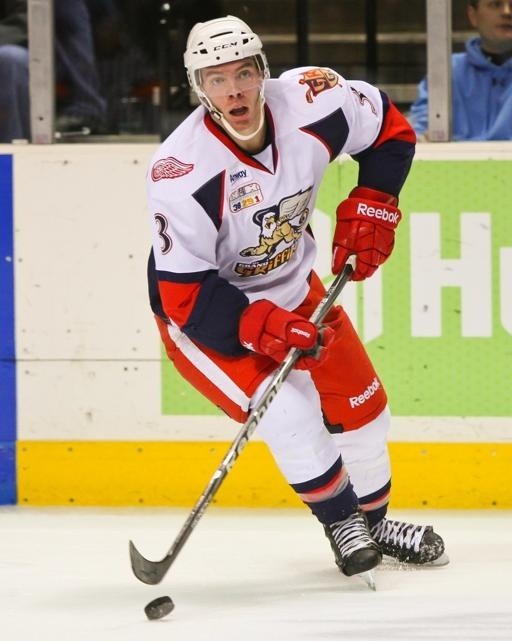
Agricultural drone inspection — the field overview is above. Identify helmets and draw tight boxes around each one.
[184,14,270,92]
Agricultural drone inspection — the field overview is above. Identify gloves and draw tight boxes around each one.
[238,298,335,369]
[331,185,401,280]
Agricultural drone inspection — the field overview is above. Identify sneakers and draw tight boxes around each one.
[325,506,382,575]
[369,517,444,564]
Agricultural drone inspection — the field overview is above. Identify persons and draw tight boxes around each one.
[148,14,445,577]
[0,1,109,142]
[410,1,512,140]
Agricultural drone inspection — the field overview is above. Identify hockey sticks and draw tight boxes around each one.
[130,256,356,588]
